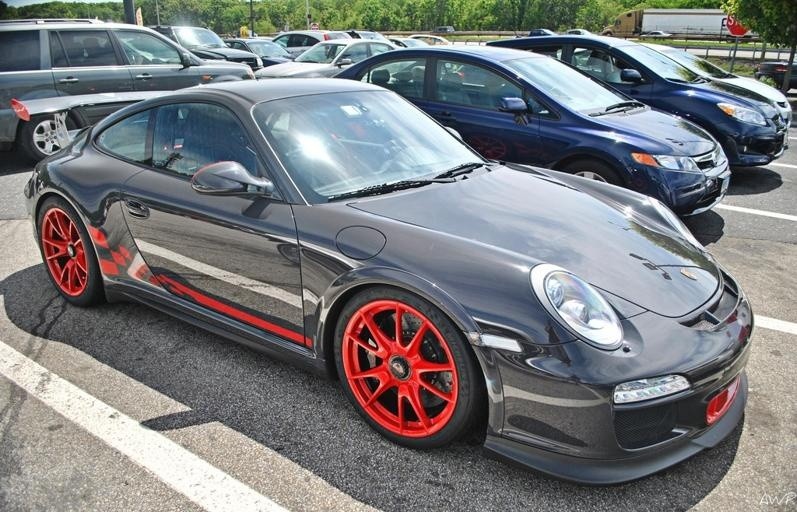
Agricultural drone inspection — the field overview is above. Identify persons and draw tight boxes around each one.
[239,24,249,39]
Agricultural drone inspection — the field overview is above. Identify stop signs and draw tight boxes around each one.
[726,14,751,37]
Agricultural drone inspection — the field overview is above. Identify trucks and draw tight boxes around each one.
[602,8,760,43]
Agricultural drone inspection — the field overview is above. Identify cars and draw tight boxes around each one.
[0,18,797,484]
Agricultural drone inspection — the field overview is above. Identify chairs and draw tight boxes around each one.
[120,110,350,198]
[372,65,518,110]
